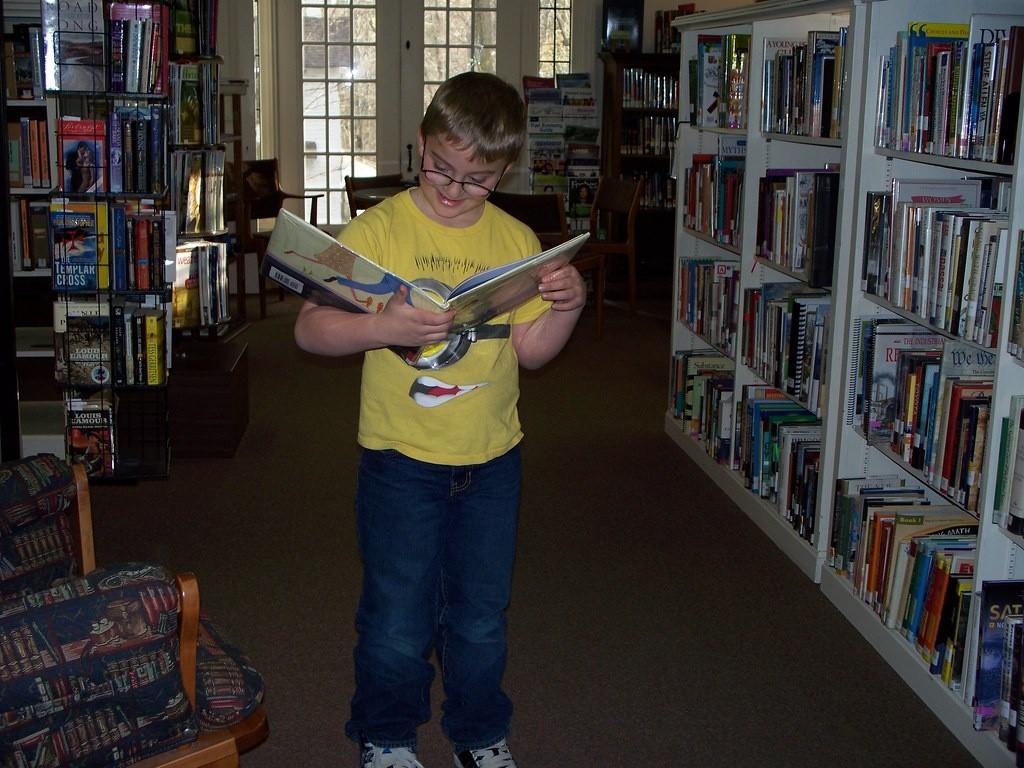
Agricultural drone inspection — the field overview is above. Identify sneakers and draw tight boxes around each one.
[361,741,425,768]
[453,737,517,768]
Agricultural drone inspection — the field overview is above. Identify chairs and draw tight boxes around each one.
[0,453,270,768]
[569,174,645,313]
[345,173,403,219]
[487,191,607,342]
[243,189,333,319]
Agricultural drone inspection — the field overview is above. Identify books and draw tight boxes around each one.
[0,0,227,479]
[655,10,706,54]
[620,67,679,208]
[669,13,1024,768]
[261,209,589,366]
[523,72,606,292]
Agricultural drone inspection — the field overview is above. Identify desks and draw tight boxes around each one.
[352,185,413,210]
[596,0,1024,768]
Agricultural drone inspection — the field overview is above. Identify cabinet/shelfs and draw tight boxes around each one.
[0,0,251,486]
[128,342,251,459]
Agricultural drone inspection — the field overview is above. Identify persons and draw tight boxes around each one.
[78,149,95,193]
[292,71,586,768]
[573,184,593,208]
[66,141,88,191]
[544,186,553,193]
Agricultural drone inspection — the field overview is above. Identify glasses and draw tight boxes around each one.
[420,137,508,197]
